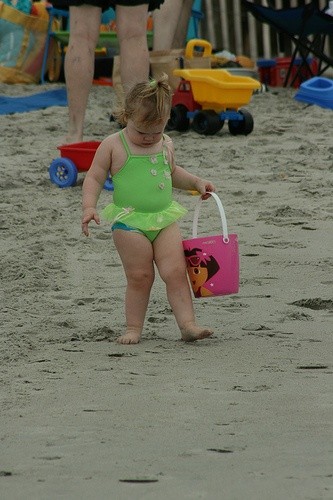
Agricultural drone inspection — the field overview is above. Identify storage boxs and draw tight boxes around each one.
[256,57,319,88]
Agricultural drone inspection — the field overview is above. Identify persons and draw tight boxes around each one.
[48,0,165,146]
[153,0,194,56]
[81,75,216,344]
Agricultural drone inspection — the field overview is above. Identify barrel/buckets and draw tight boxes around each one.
[182,191,239,297]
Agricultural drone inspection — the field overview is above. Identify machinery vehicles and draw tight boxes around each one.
[172,68,261,137]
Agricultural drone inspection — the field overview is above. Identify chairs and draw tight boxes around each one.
[242,0,333,87]
